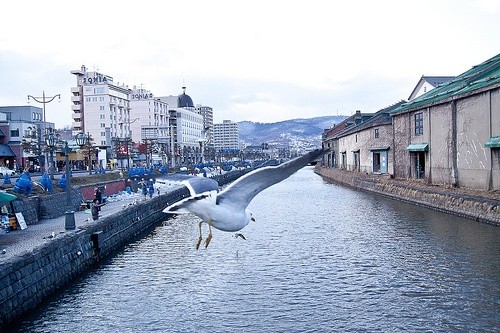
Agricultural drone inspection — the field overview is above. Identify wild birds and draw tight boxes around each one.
[52,231,56,238]
[2,248,7,254]
[144,149,327,250]
[231,232,246,241]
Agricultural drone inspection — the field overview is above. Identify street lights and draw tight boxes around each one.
[46,126,59,179]
[63,141,76,230]
[119,116,141,162]
[28,90,62,175]
[76,132,89,172]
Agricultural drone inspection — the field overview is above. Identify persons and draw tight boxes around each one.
[91,187,108,221]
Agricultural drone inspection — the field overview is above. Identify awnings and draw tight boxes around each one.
[484,136,500,147]
[340,151,346,153]
[368,146,390,151]
[351,149,360,152]
[407,144,428,152]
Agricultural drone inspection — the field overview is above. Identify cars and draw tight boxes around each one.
[0,166,16,177]
